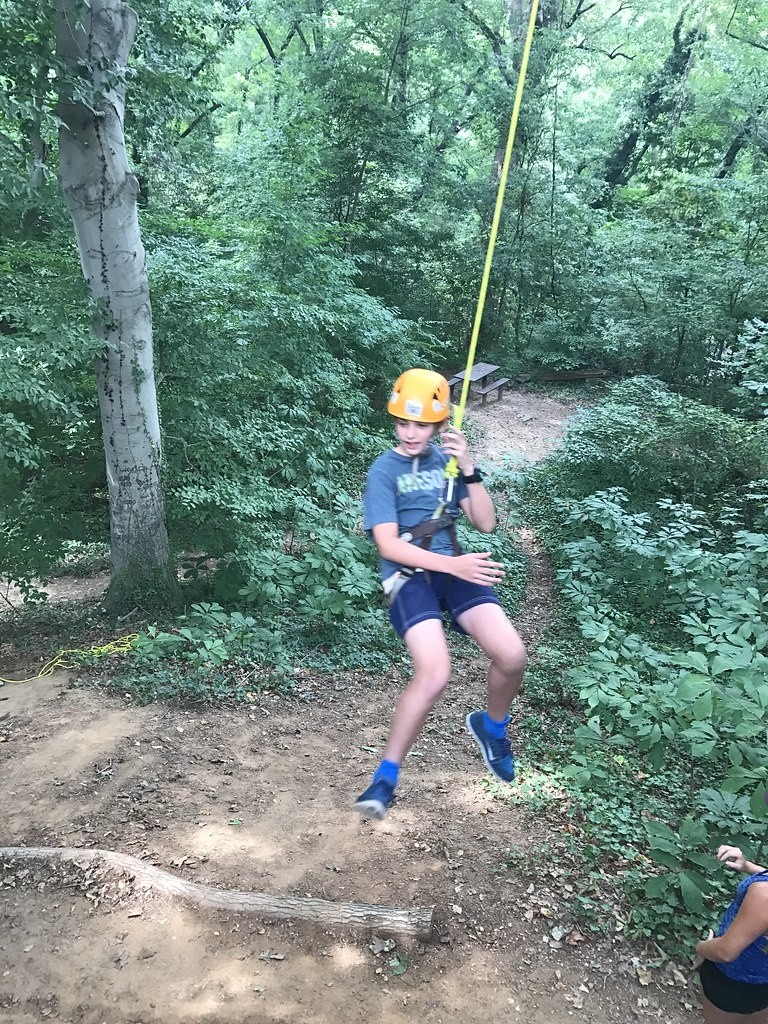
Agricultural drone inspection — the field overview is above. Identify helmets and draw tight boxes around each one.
[387,368,452,423]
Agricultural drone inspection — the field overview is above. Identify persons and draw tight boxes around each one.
[688,845,768,1024]
[351,368,527,819]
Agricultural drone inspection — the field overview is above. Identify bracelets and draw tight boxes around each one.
[457,472,485,483]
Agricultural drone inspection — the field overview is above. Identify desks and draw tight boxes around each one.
[453,362,501,400]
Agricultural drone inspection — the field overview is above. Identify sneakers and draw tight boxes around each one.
[354,778,394,820]
[465,710,514,782]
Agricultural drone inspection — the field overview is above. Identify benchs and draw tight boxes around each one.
[476,378,511,405]
[448,377,464,397]
